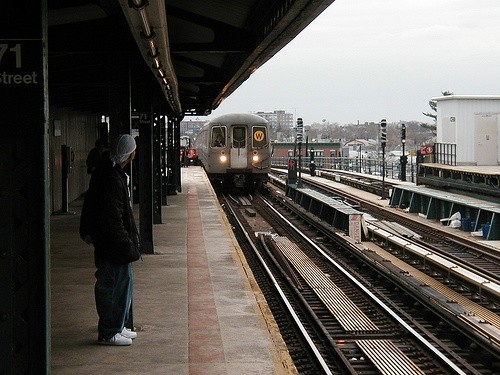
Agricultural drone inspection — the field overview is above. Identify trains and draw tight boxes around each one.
[192,112,271,196]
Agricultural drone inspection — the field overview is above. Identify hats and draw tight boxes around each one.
[109,133,137,163]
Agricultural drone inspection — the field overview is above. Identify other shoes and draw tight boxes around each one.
[97,327,137,346]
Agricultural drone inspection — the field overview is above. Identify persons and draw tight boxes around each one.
[79,134,143,345]
[86,139,109,174]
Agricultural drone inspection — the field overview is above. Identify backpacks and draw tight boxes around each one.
[81,190,96,243]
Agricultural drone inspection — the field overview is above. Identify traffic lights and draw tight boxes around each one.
[401,124,406,144]
[309,147,315,164]
